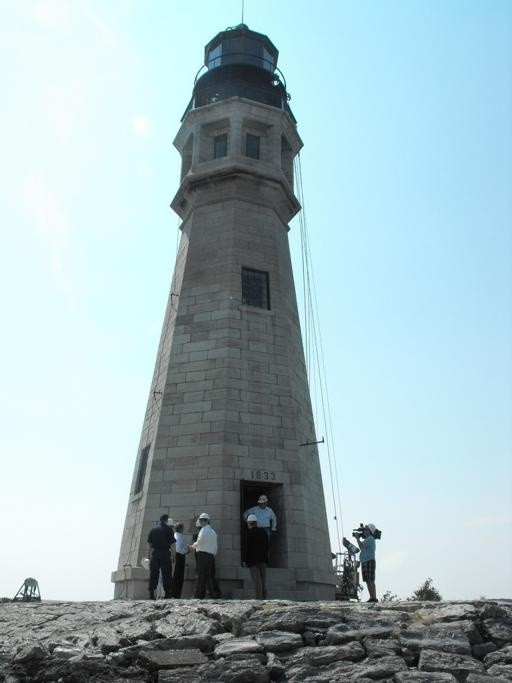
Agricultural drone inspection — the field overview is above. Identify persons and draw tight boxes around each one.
[241,513,271,599]
[188,513,222,599]
[169,522,192,598]
[147,514,177,600]
[242,495,277,568]
[355,523,379,602]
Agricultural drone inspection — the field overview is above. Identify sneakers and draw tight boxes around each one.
[367,598,379,603]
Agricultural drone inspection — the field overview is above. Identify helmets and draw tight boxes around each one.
[258,495,268,503]
[165,518,174,525]
[195,513,210,526]
[141,557,150,569]
[365,522,376,534]
[247,514,257,522]
